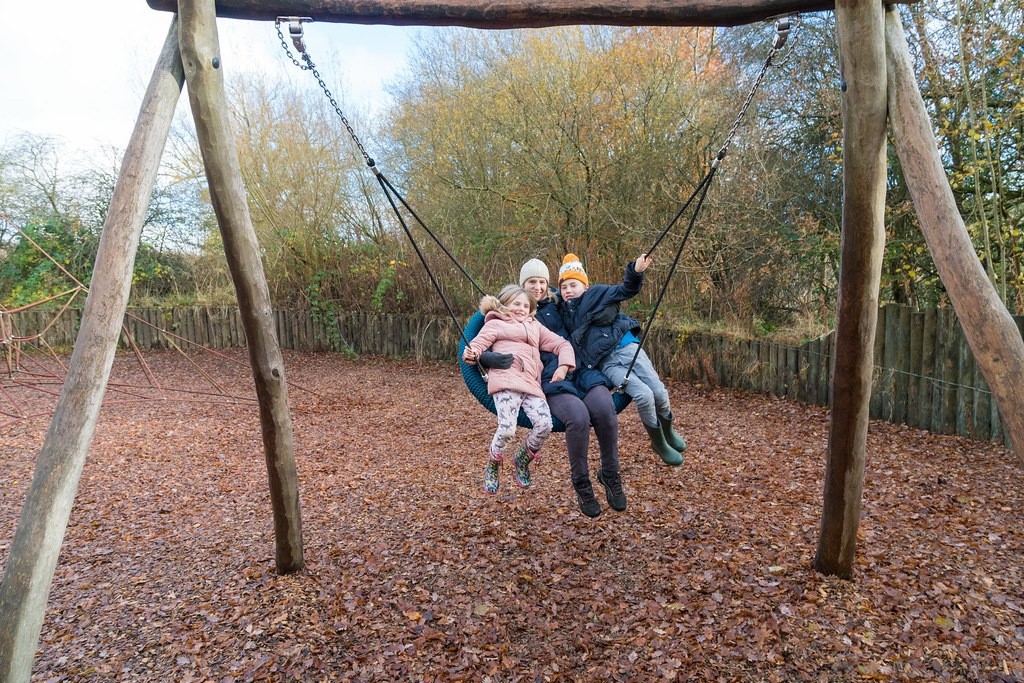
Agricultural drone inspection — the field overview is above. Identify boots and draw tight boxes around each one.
[483,446,505,495]
[513,441,542,489]
[655,409,687,452]
[643,420,684,466]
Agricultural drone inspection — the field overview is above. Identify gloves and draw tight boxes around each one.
[479,351,514,369]
[590,305,617,326]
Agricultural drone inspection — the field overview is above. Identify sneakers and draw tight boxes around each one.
[572,479,601,519]
[597,469,627,512]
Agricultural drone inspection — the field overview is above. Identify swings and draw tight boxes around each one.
[274,20,797,432]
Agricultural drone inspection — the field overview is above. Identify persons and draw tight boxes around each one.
[463,285,575,494]
[556,254,686,466]
[479,258,626,517]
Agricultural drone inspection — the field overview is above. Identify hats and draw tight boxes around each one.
[558,253,588,287]
[520,259,550,288]
